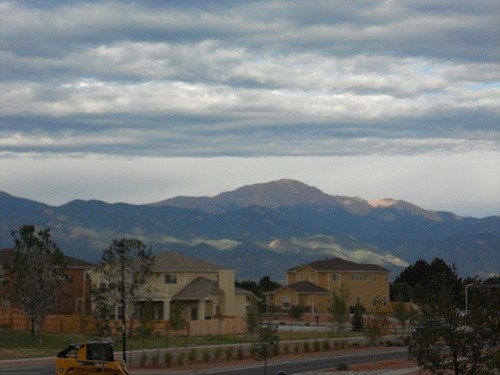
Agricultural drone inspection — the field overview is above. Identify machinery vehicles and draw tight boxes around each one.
[56,342,125,375]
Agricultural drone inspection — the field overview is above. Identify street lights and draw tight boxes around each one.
[117,240,127,364]
